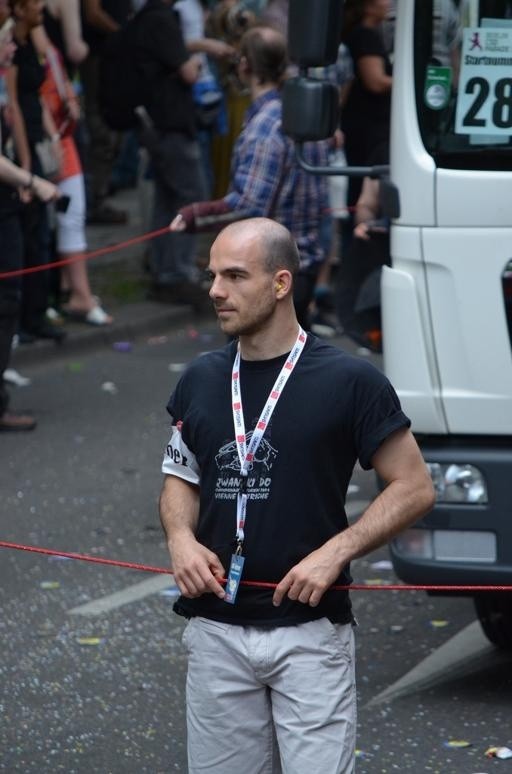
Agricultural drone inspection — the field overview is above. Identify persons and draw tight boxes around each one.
[1,2,477,436]
[155,217,440,772]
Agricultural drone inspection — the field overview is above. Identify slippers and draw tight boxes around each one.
[58,303,115,327]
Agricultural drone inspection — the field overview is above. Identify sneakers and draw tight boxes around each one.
[17,324,67,344]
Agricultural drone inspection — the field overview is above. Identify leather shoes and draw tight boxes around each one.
[0,410,36,431]
